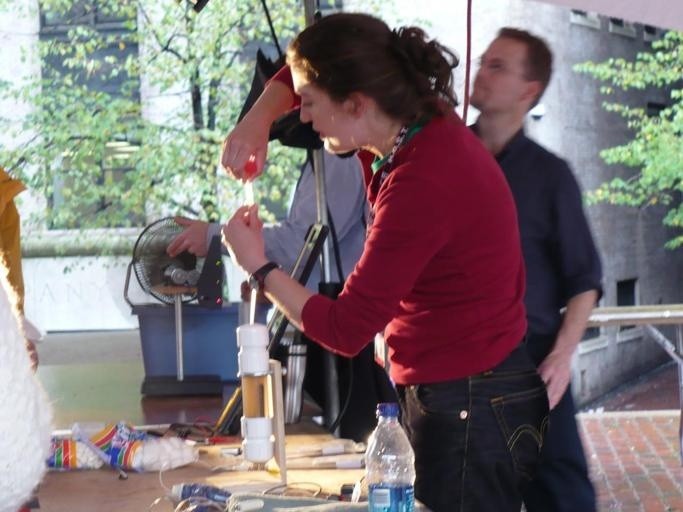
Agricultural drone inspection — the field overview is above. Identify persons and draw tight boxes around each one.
[164,146,402,448]
[219,11,551,511]
[0,168,53,511]
[465,26,606,511]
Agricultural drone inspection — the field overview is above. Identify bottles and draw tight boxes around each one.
[364,401,417,512]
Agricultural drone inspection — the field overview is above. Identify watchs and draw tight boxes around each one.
[246,258,282,292]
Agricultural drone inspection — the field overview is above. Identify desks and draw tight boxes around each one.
[37,358,432,507]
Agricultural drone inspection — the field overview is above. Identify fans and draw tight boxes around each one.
[131,218,223,396]
